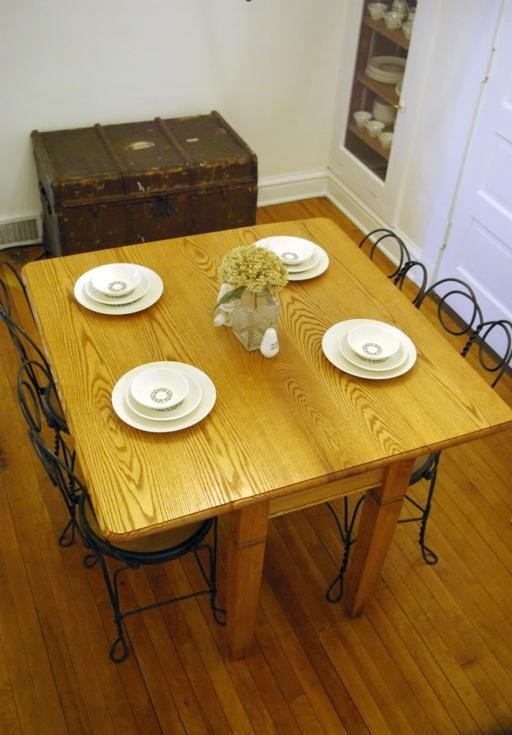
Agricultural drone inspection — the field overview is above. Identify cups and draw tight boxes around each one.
[352,111,395,150]
[367,1,418,41]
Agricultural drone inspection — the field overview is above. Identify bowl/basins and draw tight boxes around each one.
[130,373,190,410]
[273,242,315,265]
[348,329,399,362]
[91,271,143,298]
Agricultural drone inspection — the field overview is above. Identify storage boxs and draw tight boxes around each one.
[28,110,258,257]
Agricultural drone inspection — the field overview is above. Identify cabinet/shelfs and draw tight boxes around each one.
[334,0,439,225]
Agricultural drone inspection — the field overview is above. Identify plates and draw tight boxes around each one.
[360,54,405,96]
[368,98,397,125]
[320,318,418,381]
[110,359,217,434]
[71,261,165,315]
[249,235,329,283]
